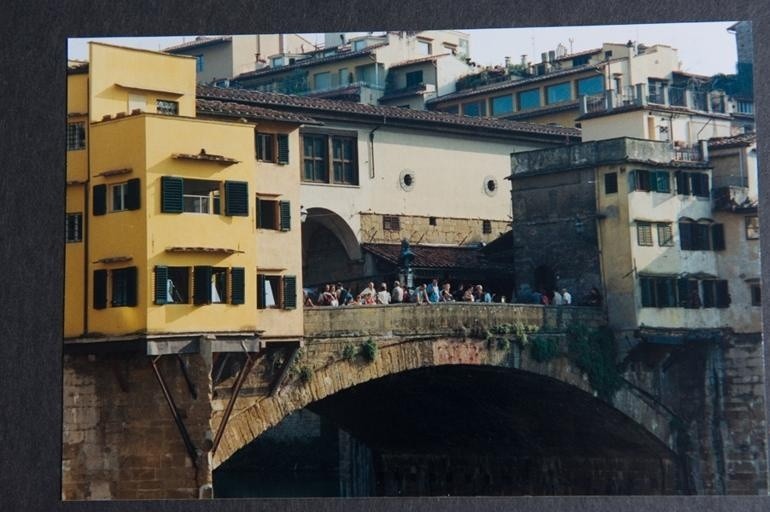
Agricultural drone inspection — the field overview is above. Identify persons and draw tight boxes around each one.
[587,286,601,304]
[303,276,573,308]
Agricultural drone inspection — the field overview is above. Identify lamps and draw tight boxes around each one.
[301,204,308,223]
